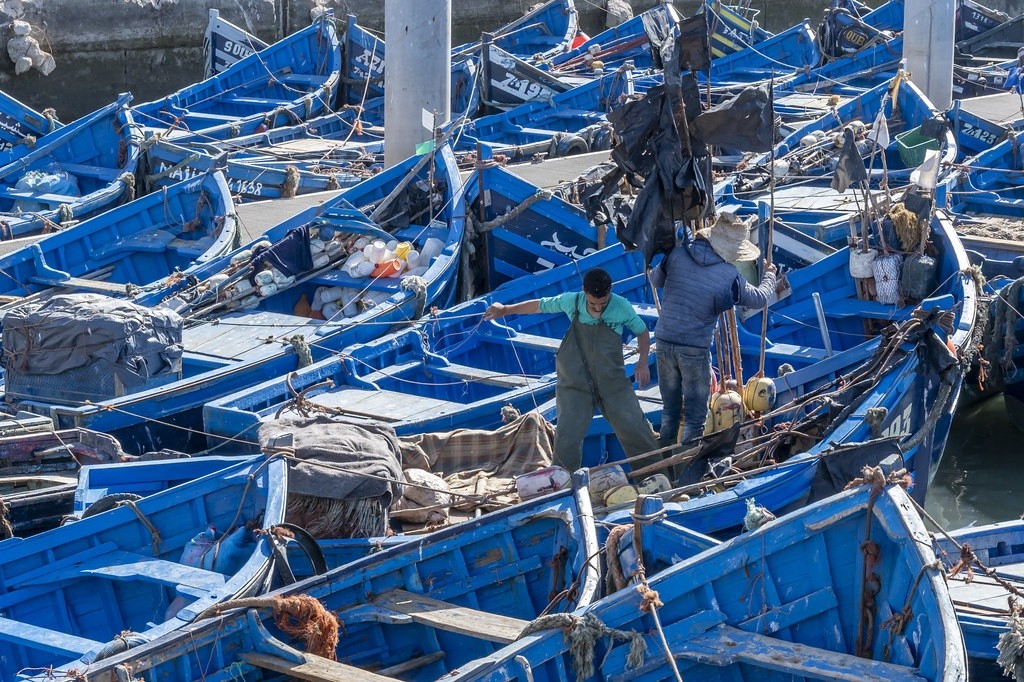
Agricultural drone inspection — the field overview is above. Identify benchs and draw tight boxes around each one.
[424,355,538,389]
[235,648,403,682]
[871,72,895,80]
[220,97,291,107]
[831,85,870,94]
[48,162,118,181]
[311,271,404,291]
[764,343,841,363]
[277,75,329,86]
[675,625,917,682]
[0,548,232,658]
[557,109,606,120]
[186,112,238,122]
[61,278,133,295]
[373,588,531,645]
[966,190,1024,212]
[735,68,793,76]
[475,139,514,149]
[507,333,562,352]
[0,185,78,204]
[518,125,574,137]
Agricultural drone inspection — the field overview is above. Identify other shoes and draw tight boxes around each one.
[670,494,691,501]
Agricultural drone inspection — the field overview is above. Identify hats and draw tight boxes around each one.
[696,212,761,262]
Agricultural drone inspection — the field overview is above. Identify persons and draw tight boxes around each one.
[484,269,670,483]
[651,211,777,471]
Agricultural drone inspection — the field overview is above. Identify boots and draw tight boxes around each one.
[672,444,704,495]
[657,436,678,480]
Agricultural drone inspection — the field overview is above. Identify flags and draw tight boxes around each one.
[867,92,890,149]
[830,131,867,194]
[910,150,941,189]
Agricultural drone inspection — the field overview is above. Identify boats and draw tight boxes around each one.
[0,0,1023,682]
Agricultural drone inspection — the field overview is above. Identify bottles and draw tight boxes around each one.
[349,262,379,278]
[321,286,343,302]
[341,235,420,270]
[369,259,406,278]
[342,286,357,315]
